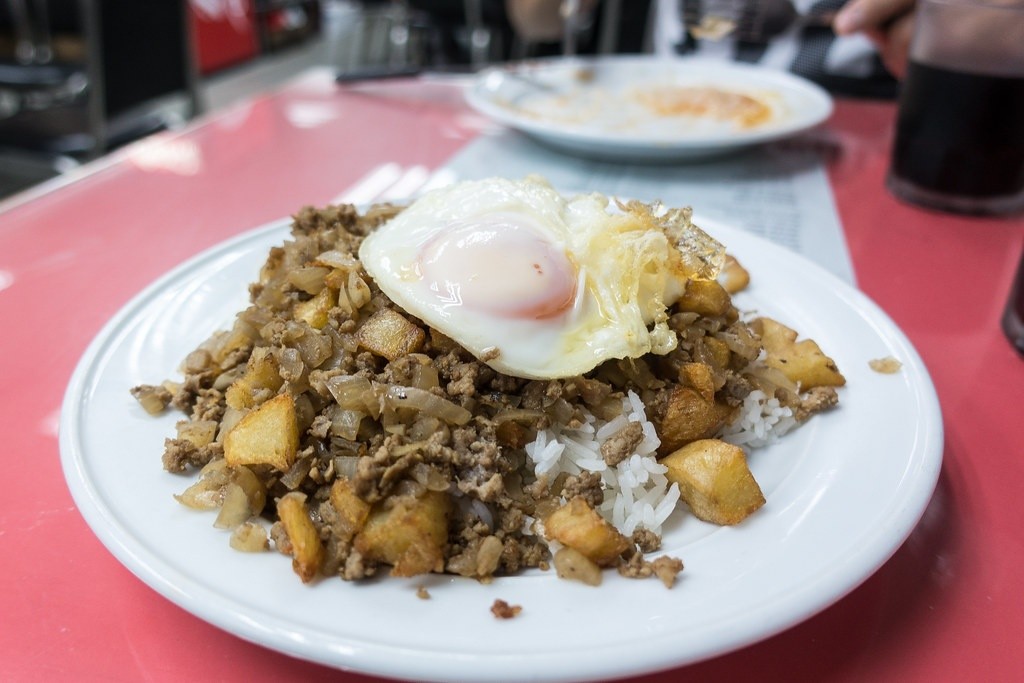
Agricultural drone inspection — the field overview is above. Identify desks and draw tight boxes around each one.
[0,65,1024,683]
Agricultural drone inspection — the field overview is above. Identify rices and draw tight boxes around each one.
[522,390,794,537]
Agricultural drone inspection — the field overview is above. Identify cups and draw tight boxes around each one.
[891,0,1024,212]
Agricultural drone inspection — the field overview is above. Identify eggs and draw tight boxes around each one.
[361,177,683,381]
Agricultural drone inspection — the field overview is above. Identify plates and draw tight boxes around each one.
[63,208,944,683]
[464,59,836,160]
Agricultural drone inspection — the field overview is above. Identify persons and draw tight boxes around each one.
[834,0,914,103]
